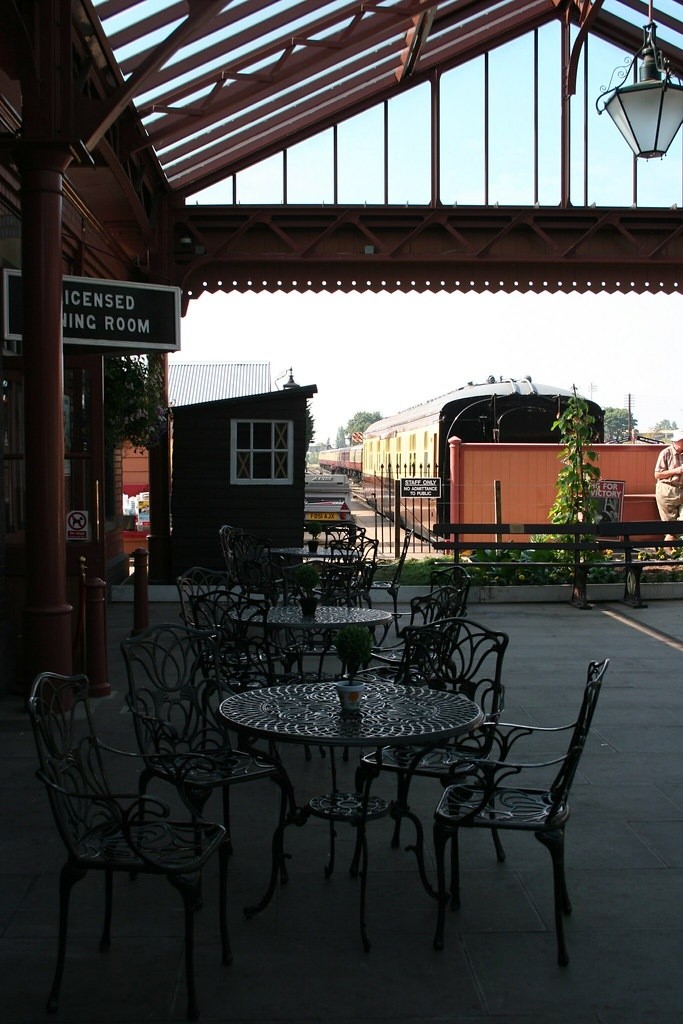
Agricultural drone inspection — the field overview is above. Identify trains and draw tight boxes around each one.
[316,442,363,482]
[358,374,607,536]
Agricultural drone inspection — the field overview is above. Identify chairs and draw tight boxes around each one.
[27,523,609,1024]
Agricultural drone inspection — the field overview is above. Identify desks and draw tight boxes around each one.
[229,605,394,760]
[265,547,363,557]
[215,681,485,950]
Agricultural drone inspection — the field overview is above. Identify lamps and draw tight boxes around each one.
[595,0,683,163]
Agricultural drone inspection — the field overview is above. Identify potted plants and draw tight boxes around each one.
[334,623,373,710]
[304,520,323,552]
[295,564,319,615]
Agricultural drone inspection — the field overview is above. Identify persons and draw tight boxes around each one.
[653,429,683,554]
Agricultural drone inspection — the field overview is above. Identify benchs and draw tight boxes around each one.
[433,520,683,609]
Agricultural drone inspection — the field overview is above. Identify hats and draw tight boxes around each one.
[670,430,683,442]
